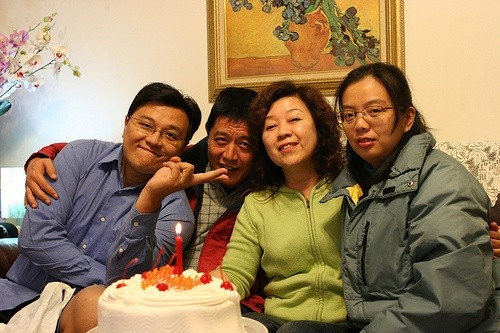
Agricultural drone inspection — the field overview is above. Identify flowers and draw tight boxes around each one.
[0,10,82,108]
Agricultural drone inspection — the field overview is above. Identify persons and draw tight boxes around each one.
[0,82,227,333]
[207,80,500,333]
[23,87,267,313]
[320,63,500,333]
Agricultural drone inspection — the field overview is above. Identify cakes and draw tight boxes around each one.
[96,250,245,333]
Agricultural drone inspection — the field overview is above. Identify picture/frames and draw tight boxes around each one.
[206,0,407,103]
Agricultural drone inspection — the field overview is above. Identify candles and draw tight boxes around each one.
[174,223,184,274]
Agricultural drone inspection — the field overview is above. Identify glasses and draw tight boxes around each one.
[127,115,188,148]
[335,105,408,124]
